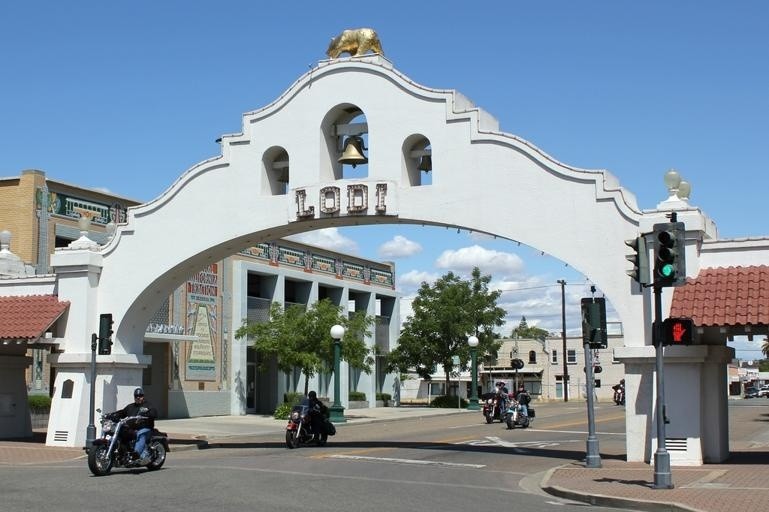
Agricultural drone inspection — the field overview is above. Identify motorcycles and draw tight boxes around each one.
[86,407,170,477]
[478,386,535,430]
[610,380,625,406]
[285,399,333,448]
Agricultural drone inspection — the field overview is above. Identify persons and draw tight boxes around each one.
[491,382,508,412]
[515,383,528,423]
[308,391,328,446]
[614,379,625,388]
[104,388,158,465]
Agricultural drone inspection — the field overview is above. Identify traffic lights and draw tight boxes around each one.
[97,312,115,356]
[652,222,687,290]
[625,234,649,288]
[662,317,692,346]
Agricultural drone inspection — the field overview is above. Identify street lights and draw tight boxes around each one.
[465,334,482,411]
[326,324,349,424]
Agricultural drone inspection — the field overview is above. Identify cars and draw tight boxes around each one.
[742,384,769,400]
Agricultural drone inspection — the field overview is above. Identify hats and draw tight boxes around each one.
[133,388,144,396]
